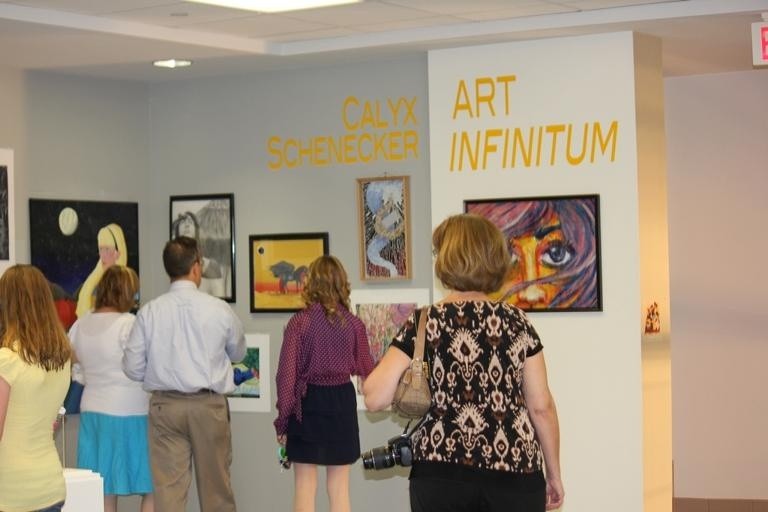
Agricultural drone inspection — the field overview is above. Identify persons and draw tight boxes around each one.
[0,259,73,511]
[362,212,566,511]
[121,235,248,511]
[175,212,225,299]
[272,255,375,512]
[65,263,152,510]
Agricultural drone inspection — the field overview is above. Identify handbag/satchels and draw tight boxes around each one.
[392,361,432,418]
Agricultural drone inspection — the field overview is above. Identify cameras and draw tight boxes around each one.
[362,432,416,470]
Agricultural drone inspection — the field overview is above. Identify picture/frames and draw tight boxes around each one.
[226,332,271,414]
[169,194,236,302]
[0,147,16,274]
[249,232,329,313]
[357,175,412,281]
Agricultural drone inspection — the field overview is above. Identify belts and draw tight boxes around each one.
[202,388,215,394]
